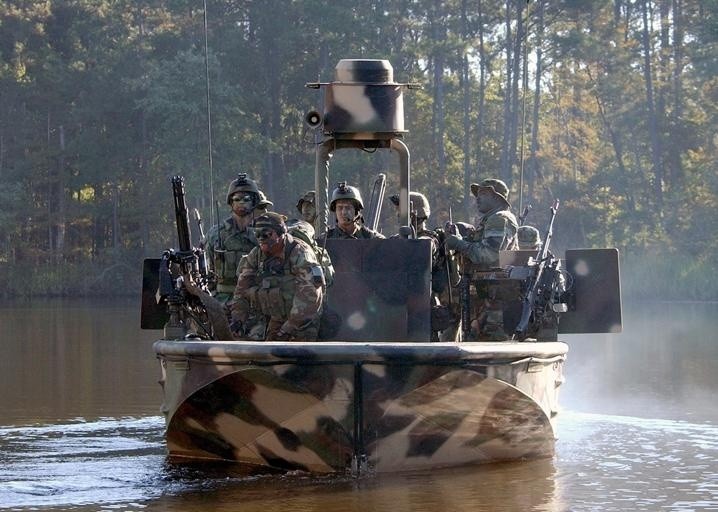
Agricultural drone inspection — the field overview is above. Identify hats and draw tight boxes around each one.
[253,212,286,236]
[296,191,315,213]
[471,178,510,207]
[256,190,273,206]
[518,226,543,246]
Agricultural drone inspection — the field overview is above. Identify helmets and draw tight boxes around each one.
[330,186,365,212]
[389,191,430,218]
[227,173,259,204]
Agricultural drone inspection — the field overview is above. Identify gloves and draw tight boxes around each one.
[229,320,245,335]
[277,329,296,341]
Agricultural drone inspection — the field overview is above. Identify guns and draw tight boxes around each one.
[512,198,560,342]
[459,258,471,331]
[163,174,215,325]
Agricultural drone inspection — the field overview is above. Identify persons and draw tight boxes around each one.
[252,191,272,219]
[199,171,269,339]
[297,187,328,231]
[518,223,560,342]
[445,178,523,342]
[307,184,388,239]
[391,190,445,343]
[226,211,324,342]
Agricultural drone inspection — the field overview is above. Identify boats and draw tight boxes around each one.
[136,58,627,477]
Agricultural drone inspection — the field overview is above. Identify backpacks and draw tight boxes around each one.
[285,219,335,289]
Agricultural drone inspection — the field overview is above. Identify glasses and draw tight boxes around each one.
[232,196,253,202]
[257,205,268,209]
[253,231,272,238]
